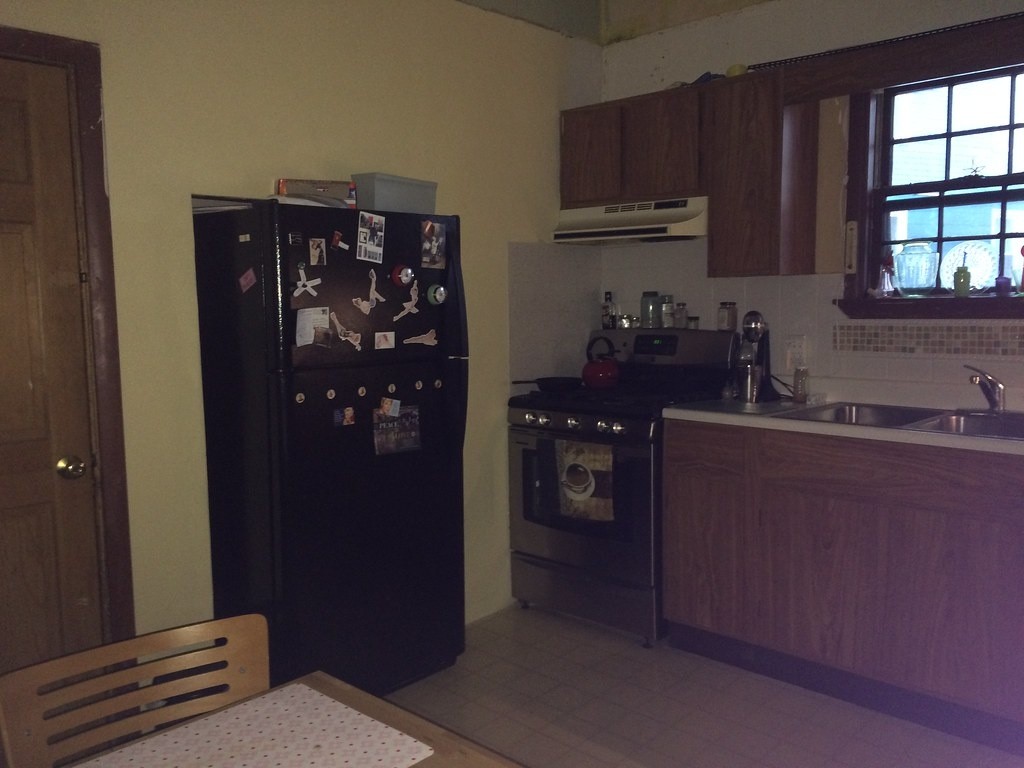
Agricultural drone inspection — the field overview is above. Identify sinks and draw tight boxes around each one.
[914,413,1024,439]
[770,402,947,430]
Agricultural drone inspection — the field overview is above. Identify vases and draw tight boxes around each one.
[876,266,895,298]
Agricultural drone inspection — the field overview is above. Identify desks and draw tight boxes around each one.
[61,669,528,768]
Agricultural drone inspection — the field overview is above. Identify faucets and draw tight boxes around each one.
[963,364,1006,411]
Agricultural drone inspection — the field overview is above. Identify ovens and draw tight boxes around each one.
[507,407,669,643]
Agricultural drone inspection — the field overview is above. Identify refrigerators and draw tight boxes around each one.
[192,205,469,697]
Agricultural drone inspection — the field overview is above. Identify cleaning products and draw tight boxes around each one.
[792,367,809,406]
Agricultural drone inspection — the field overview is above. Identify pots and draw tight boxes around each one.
[512,376,582,392]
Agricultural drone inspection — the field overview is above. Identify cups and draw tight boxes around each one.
[734,365,762,403]
[996,276,1010,296]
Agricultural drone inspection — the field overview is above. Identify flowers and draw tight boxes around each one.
[880,246,895,276]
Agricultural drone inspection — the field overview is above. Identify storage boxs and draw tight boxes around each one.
[278,173,437,215]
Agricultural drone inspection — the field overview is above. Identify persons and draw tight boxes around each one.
[369,222,378,245]
[380,397,393,415]
[343,407,355,425]
[423,237,439,263]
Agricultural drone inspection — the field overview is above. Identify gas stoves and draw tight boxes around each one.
[507,391,721,418]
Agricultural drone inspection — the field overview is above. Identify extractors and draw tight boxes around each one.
[551,196,709,244]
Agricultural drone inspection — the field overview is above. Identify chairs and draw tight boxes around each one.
[0,613,268,768]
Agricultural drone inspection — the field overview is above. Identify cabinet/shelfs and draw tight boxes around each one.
[662,418,1024,754]
[558,67,816,279]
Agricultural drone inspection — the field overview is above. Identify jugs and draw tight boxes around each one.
[583,336,624,391]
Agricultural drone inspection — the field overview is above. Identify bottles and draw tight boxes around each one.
[737,335,757,363]
[896,240,940,298]
[875,266,895,298]
[601,291,699,328]
[718,302,737,331]
[954,267,970,298]
[794,367,808,403]
